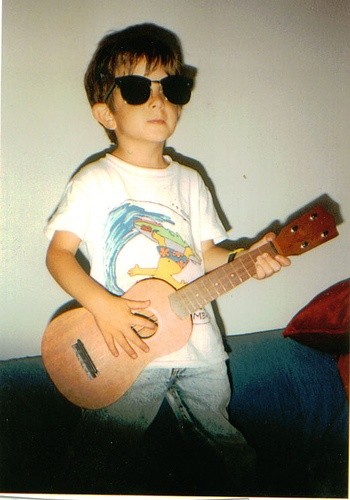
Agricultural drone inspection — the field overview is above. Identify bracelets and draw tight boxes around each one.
[226,245,246,262]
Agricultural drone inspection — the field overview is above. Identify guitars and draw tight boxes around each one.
[39,201,342,412]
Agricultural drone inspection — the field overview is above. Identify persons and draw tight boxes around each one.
[44,23,291,481]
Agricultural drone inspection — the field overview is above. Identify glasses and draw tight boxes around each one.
[103,75,194,105]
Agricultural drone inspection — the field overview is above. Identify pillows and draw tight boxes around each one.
[283,279,350,353]
